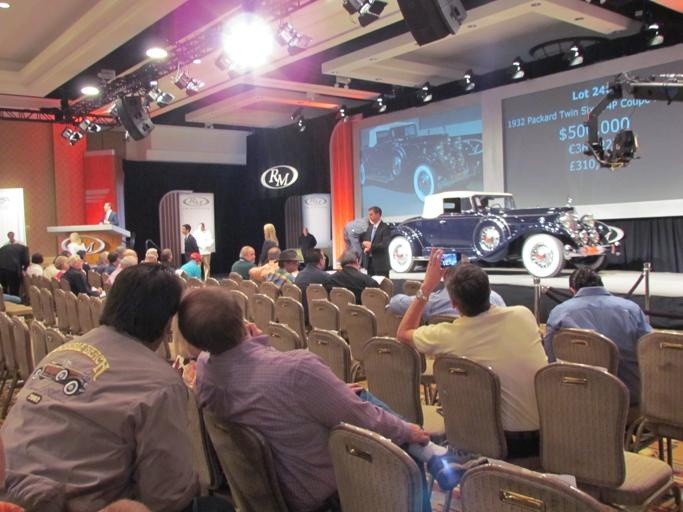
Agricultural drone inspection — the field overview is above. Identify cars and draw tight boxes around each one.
[358,124,483,204]
[387,191,626,274]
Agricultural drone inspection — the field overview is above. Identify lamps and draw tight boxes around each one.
[61,0,387,148]
[290,20,683,133]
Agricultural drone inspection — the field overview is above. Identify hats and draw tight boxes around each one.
[274,249,304,263]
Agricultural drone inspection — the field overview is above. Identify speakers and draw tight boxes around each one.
[396,0,468,46]
[117,95,155,141]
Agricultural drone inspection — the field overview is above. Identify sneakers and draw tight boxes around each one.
[428,445,488,490]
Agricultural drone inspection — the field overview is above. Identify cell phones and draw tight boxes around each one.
[440,252,462,268]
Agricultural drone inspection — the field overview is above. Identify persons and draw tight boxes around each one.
[479,196,489,215]
[4,231,22,245]
[181,224,200,262]
[179,252,201,280]
[541,267,654,408]
[197,222,214,281]
[360,206,393,282]
[389,286,504,318]
[0,243,173,297]
[177,287,487,511]
[0,262,232,512]
[232,245,380,320]
[394,242,549,460]
[66,232,94,256]
[257,223,280,267]
[99,202,119,227]
[297,226,317,258]
